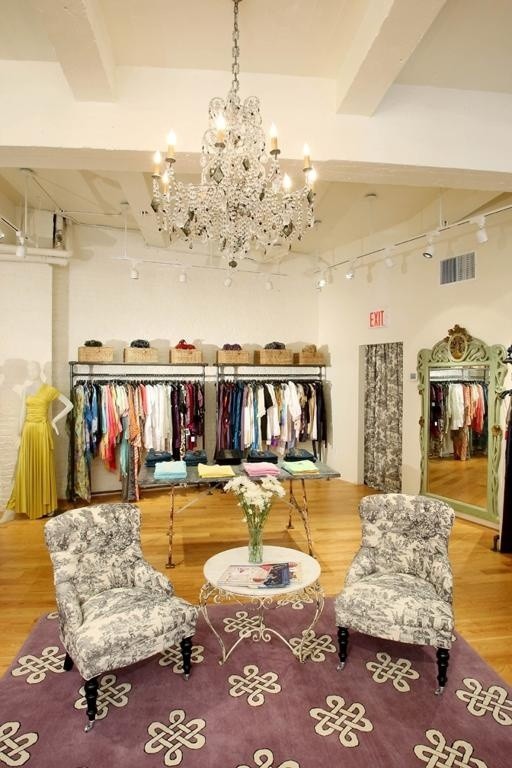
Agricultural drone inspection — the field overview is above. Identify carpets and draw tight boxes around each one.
[0,599,510,768]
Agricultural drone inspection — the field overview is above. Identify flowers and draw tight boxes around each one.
[218,473,287,530]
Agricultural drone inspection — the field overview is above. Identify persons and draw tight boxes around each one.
[0,360,74,522]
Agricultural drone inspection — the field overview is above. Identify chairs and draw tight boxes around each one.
[42,500,196,723]
[333,492,456,686]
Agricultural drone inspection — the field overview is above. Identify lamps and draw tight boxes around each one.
[1,1,489,290]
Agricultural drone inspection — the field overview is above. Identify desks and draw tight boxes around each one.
[137,450,340,569]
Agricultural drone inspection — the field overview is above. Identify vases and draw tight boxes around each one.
[245,527,267,563]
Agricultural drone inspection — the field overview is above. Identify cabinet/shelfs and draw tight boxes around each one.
[68,360,329,507]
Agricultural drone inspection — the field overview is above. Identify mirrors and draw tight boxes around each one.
[417,323,508,525]
